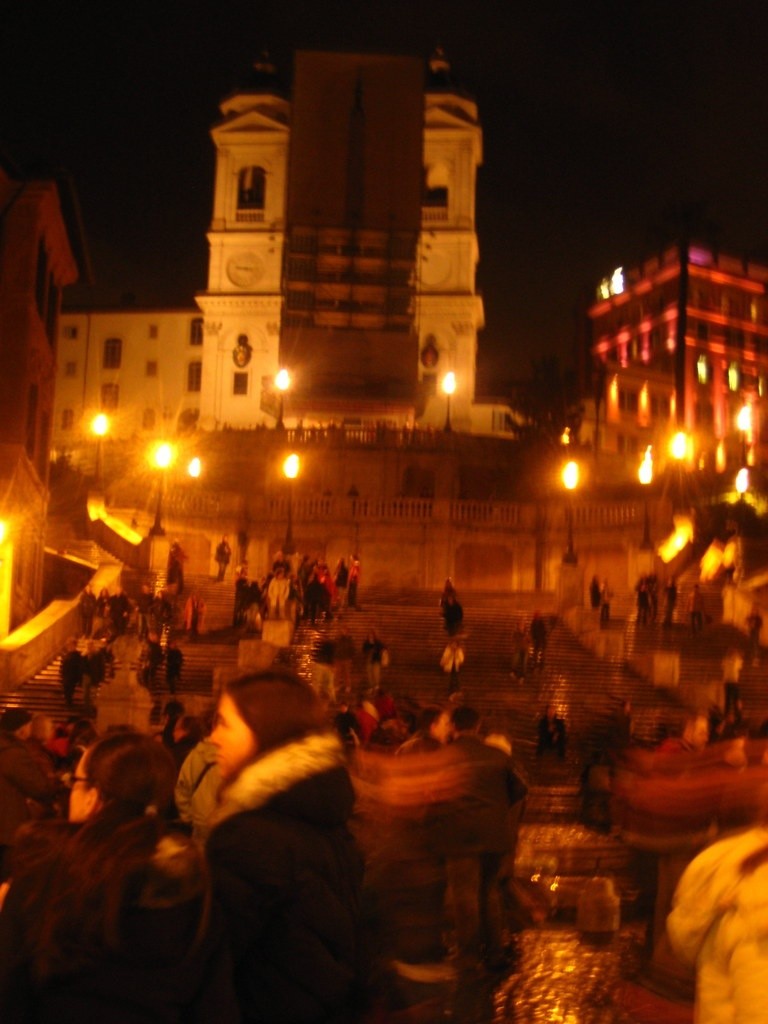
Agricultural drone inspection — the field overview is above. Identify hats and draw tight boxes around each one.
[1,707,33,731]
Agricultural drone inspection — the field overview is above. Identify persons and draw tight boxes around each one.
[0,536,767,1024]
[0,734,242,1023]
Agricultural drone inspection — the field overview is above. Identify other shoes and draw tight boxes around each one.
[449,945,482,971]
[476,949,509,978]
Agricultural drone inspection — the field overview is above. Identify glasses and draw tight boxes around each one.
[59,771,87,789]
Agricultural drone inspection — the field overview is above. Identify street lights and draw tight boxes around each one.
[280,454,302,556]
[274,368,292,431]
[441,372,458,430]
[637,457,658,552]
[148,438,179,538]
[560,462,584,565]
[90,412,111,480]
